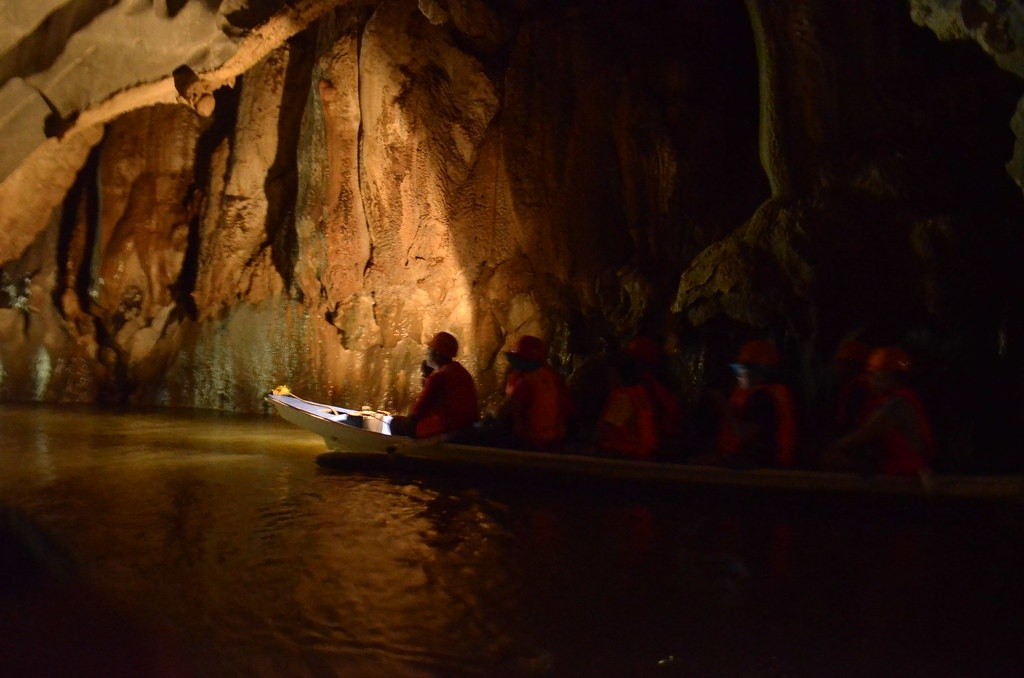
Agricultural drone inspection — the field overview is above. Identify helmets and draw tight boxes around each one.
[836,339,870,360]
[427,332,459,358]
[627,337,658,364]
[516,335,545,362]
[865,347,912,373]
[737,341,776,367]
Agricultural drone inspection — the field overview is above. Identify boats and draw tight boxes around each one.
[264,382,1024,507]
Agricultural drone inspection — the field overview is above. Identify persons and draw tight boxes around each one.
[390,332,940,473]
